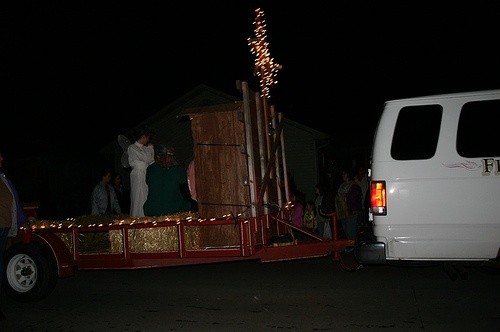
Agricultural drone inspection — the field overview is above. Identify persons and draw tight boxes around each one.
[90,123,193,217]
[0,156,35,323]
[282,169,380,272]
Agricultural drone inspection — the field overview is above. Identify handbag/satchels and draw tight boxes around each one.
[323,222,332,240]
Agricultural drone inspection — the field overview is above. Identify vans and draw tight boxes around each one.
[353,87,500,275]
